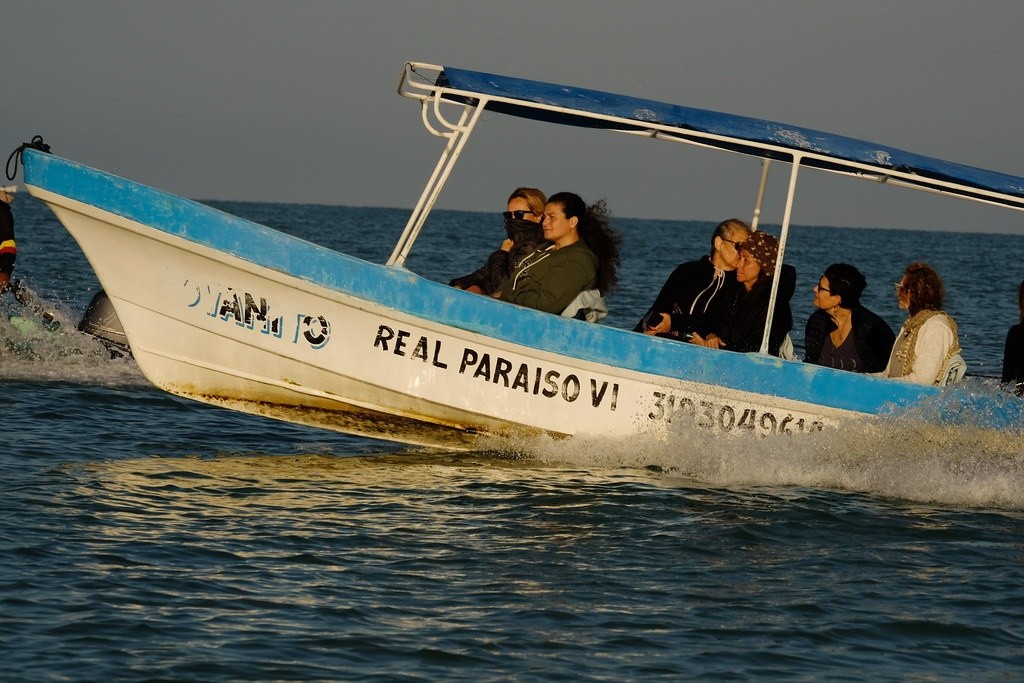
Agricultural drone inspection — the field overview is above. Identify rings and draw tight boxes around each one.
[659,328,661,333]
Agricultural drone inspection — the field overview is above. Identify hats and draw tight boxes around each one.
[824,263,867,302]
[741,230,780,278]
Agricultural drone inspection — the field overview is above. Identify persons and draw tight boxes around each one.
[631,218,962,390]
[449,185,625,315]
[1000,282,1024,397]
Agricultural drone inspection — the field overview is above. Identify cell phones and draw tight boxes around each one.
[646,311,664,327]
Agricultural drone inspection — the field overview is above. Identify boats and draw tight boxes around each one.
[23,60,1024,511]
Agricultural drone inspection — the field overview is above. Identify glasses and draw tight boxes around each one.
[894,282,902,291]
[818,275,830,293]
[725,239,741,251]
[502,210,533,220]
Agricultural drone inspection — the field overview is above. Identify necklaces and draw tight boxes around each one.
[837,313,849,340]
